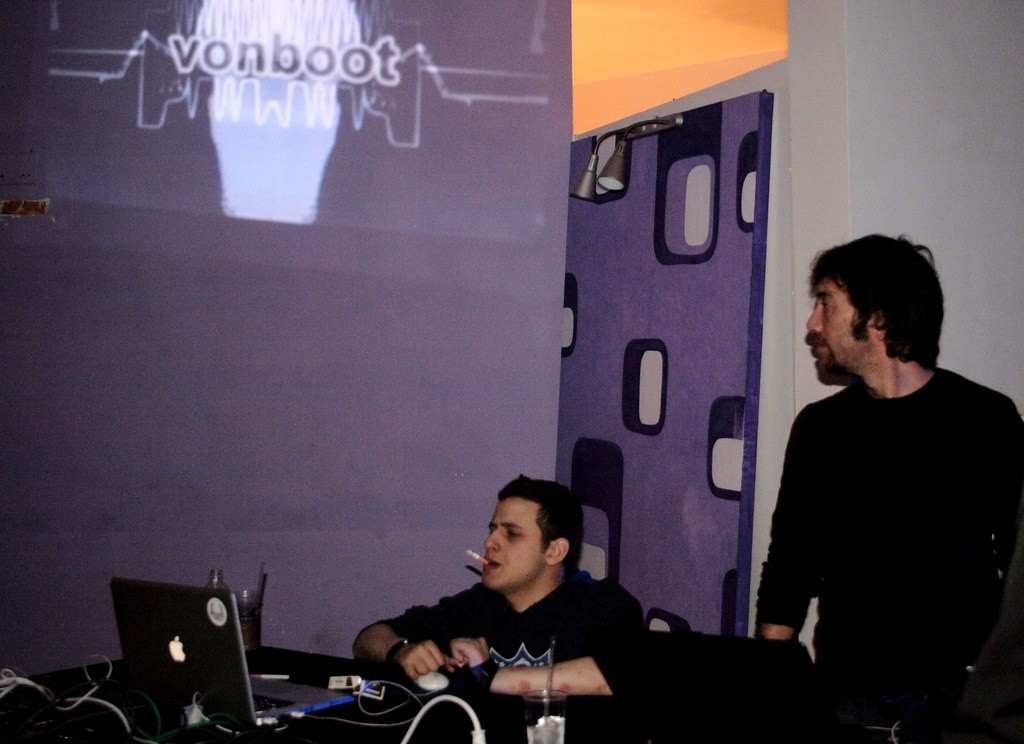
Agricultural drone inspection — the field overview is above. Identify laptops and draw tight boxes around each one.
[110,576,355,727]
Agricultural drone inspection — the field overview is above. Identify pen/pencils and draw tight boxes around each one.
[256,561,269,598]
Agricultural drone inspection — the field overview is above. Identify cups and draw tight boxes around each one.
[523,690,566,744]
[235,590,263,651]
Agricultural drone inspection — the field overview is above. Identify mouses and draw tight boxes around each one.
[414,672,450,691]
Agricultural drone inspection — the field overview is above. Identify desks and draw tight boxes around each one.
[0,647,1024,744]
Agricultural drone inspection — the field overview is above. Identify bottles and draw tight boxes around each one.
[205,568,230,593]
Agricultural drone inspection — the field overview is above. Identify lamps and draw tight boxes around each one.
[572,112,683,200]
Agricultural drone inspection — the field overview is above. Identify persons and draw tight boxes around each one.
[351,473,655,698]
[757,235,1024,744]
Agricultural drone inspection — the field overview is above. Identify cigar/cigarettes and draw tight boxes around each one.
[464,550,489,566]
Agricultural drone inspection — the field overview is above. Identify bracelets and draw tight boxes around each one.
[384,639,415,665]
